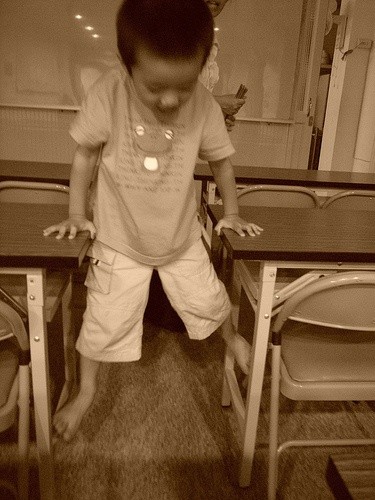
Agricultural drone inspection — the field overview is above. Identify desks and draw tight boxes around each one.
[193,163,375,191]
[0,203,96,499]
[1,159,98,185]
[207,203,375,488]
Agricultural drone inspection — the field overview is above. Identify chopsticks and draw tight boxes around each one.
[224,84,248,122]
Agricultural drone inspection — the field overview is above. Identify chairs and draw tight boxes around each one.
[242,271,375,500]
[1,300,29,460]
[321,191,374,206]
[0,182,69,203]
[235,184,319,208]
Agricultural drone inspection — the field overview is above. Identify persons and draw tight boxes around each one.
[143,0,247,333]
[41,0,264,440]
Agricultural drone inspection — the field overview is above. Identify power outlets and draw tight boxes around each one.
[357,40,374,49]
